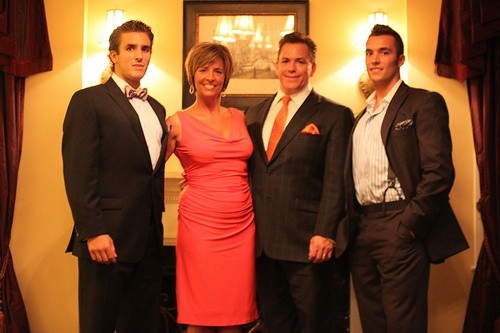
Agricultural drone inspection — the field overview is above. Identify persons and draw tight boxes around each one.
[63,20,169,333]
[162,40,257,333]
[344,24,470,333]
[171,33,356,333]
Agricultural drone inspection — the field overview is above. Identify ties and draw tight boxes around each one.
[266,96,292,162]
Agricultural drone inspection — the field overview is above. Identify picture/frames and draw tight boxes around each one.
[181,0,310,114]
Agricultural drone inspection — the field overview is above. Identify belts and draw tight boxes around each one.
[359,201,401,213]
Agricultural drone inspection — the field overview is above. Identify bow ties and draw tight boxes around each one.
[125,86,148,102]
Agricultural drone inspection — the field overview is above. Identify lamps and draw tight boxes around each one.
[367,10,391,32]
[105,8,125,33]
